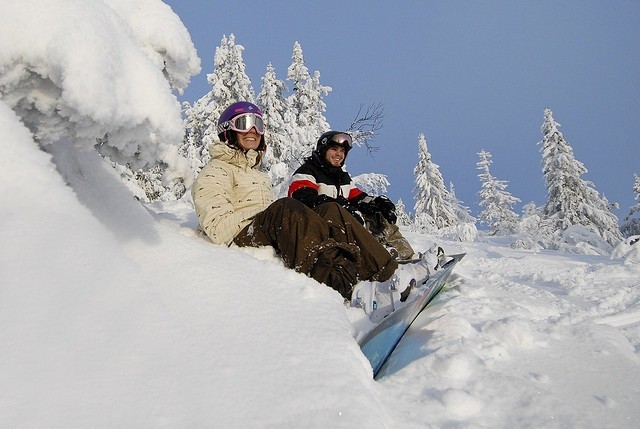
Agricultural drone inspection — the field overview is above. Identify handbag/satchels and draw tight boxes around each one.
[299,238,360,300]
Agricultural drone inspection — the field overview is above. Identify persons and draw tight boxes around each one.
[287,131,455,272]
[191,101,439,325]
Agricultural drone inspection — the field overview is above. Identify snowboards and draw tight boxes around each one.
[396,253,466,264]
[356,255,457,381]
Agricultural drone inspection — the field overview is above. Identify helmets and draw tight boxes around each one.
[316,131,352,168]
[217,101,267,152]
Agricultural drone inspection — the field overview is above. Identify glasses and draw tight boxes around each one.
[318,132,352,148]
[217,113,265,135]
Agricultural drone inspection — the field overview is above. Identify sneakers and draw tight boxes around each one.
[382,242,398,259]
[386,254,438,288]
[410,245,445,265]
[350,263,417,324]
